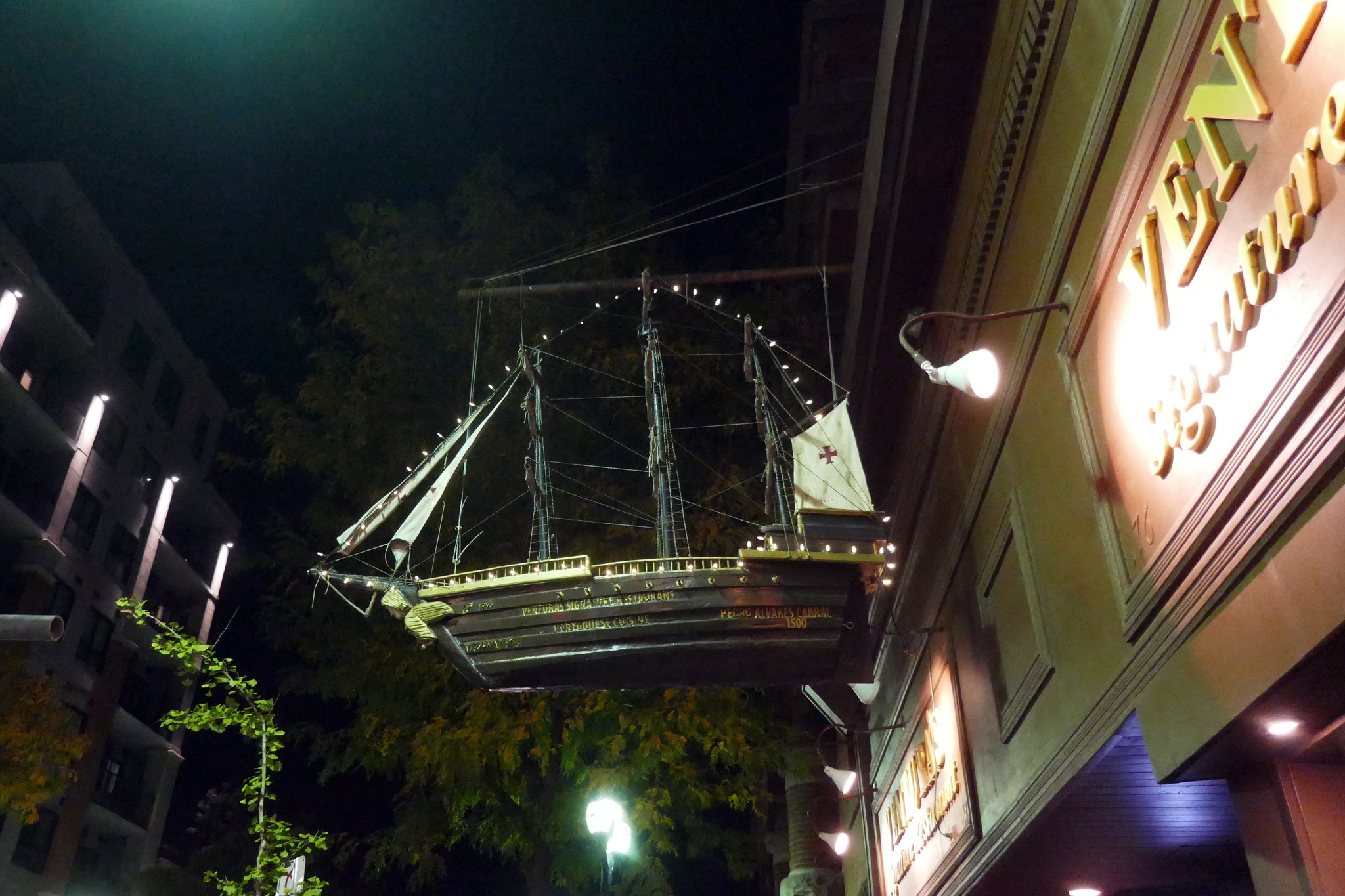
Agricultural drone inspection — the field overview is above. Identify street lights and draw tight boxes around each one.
[582,795,632,896]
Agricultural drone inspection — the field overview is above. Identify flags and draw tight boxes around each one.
[788,398,876,514]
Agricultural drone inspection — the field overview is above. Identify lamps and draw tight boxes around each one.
[895,295,1084,404]
[804,796,883,852]
[812,713,906,801]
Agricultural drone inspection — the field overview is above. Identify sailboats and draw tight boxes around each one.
[304,267,891,694]
